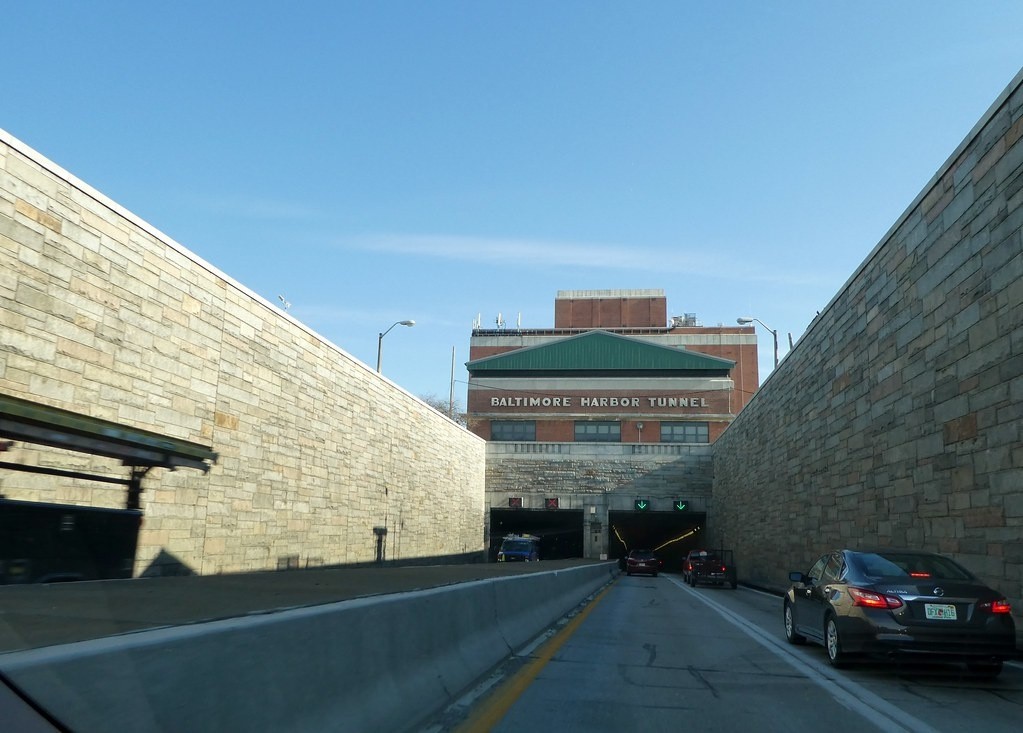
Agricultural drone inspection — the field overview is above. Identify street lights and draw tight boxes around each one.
[279,295,291,310]
[377,320,416,374]
[736,316,779,370]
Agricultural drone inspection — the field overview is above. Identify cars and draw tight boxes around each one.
[683,549,738,588]
[784,546,1017,682]
[657,553,671,571]
[624,549,658,578]
[497,537,540,562]
